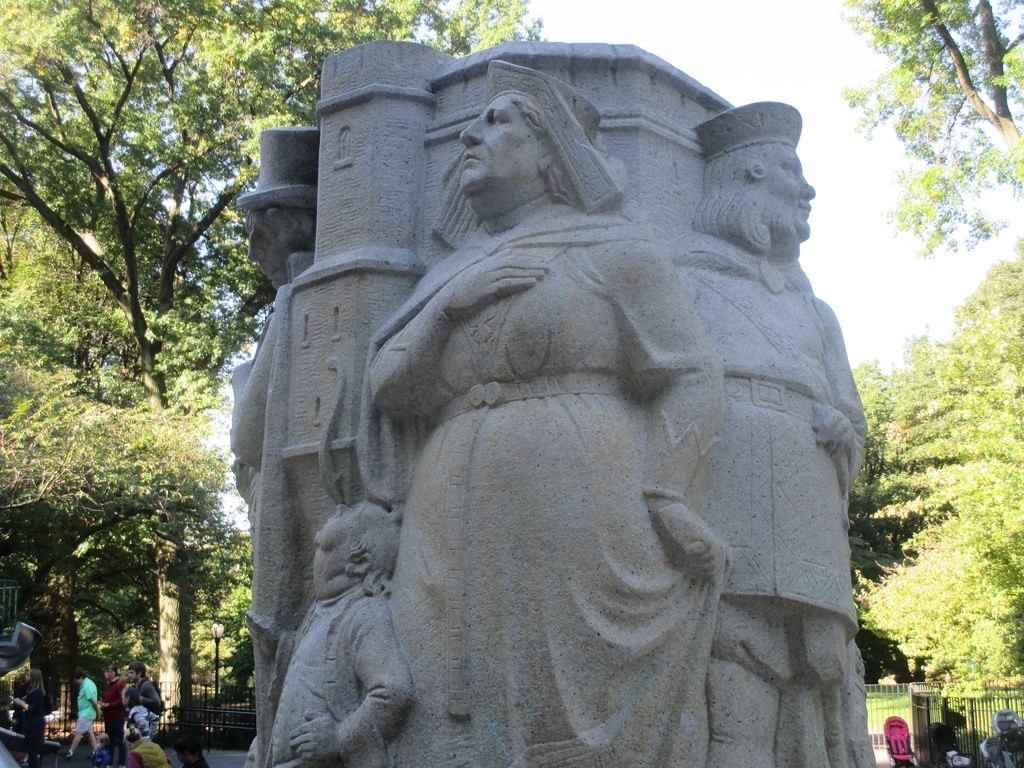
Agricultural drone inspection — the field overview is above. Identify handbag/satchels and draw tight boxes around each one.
[44,694,52,716]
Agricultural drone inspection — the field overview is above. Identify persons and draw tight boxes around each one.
[128,661,161,738]
[13,668,47,768]
[174,735,209,768]
[124,727,171,768]
[229,126,318,768]
[265,501,414,768]
[91,733,112,768]
[0,696,13,729]
[121,688,159,768]
[674,99,870,768]
[98,665,127,768]
[59,671,99,760]
[356,59,735,768]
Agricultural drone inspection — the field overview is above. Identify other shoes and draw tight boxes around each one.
[60,750,72,761]
[86,751,98,760]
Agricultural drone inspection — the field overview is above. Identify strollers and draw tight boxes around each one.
[883,716,921,768]
[926,723,978,768]
[980,709,1024,768]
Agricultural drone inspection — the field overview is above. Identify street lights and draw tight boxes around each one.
[211,621,224,707]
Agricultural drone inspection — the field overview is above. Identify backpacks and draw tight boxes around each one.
[151,681,164,714]
